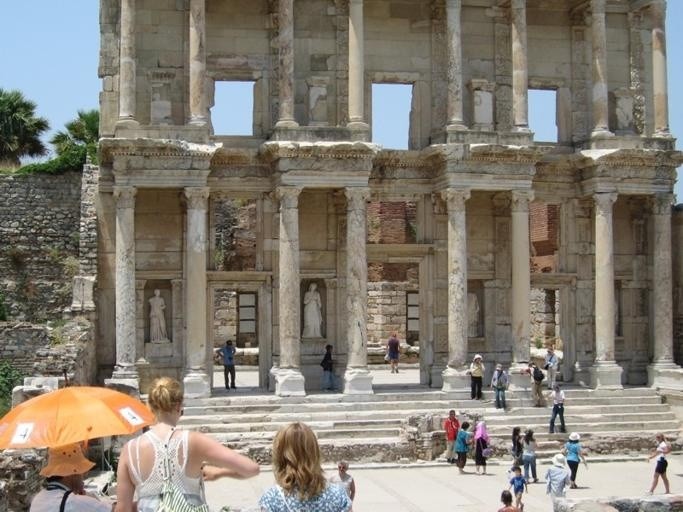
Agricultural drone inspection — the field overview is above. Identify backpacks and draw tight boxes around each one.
[532,367,543,381]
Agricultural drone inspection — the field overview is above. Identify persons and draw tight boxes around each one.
[442,410,460,463]
[386,333,400,373]
[215,340,238,390]
[258,422,351,511]
[465,293,480,334]
[148,288,168,343]
[114,375,258,511]
[471,421,488,474]
[467,346,567,434]
[320,344,338,392]
[29,445,138,511]
[303,282,322,337]
[328,460,355,502]
[647,432,671,494]
[497,425,587,511]
[453,421,473,473]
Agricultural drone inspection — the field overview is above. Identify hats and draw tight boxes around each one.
[569,432,580,441]
[547,347,554,353]
[38,443,96,478]
[551,454,565,466]
[473,354,483,361]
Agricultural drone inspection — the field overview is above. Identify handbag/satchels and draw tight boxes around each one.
[544,364,549,370]
[482,448,492,457]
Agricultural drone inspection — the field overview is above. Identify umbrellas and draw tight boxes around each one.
[0,386,156,449]
